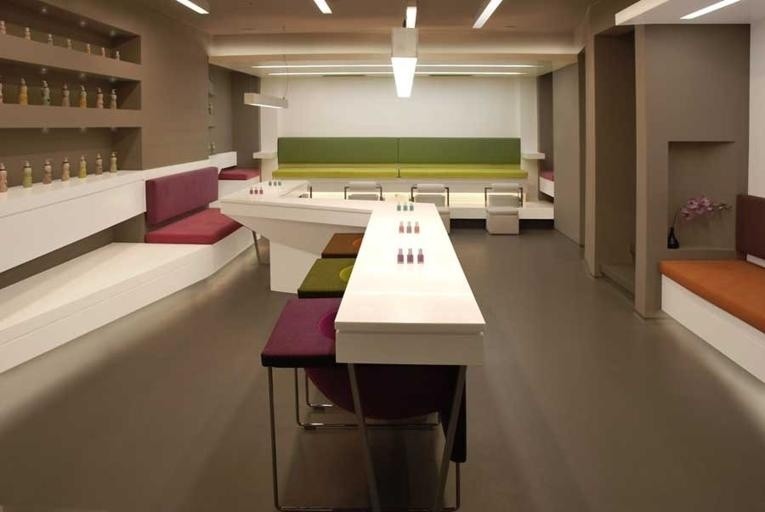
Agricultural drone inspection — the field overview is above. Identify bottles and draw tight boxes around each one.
[41,79,51,105]
[1,19,121,59]
[79,155,86,177]
[110,152,118,172]
[19,77,29,105]
[95,152,103,175]
[0,75,7,103]
[43,160,52,184]
[249,185,263,194]
[1,163,8,192]
[397,247,423,264]
[62,81,71,106]
[80,85,88,106]
[397,201,414,211]
[62,157,70,181]
[269,180,281,185]
[399,220,420,233]
[96,87,104,107]
[23,160,32,187]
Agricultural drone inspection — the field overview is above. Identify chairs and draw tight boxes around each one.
[299,182,523,235]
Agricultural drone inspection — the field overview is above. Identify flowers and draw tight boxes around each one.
[672,194,731,222]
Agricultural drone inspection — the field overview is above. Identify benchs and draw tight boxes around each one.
[660,194,765,384]
[144,150,261,279]
[277,136,528,182]
[538,169,555,202]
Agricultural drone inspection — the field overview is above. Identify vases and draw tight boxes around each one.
[666,226,683,250]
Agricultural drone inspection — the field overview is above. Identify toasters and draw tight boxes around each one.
[111,87,117,108]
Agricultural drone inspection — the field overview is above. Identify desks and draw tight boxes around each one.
[208,179,489,512]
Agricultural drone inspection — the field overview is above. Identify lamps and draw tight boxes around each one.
[243,93,288,109]
[391,28,418,100]
[175,0,210,15]
[471,0,503,29]
